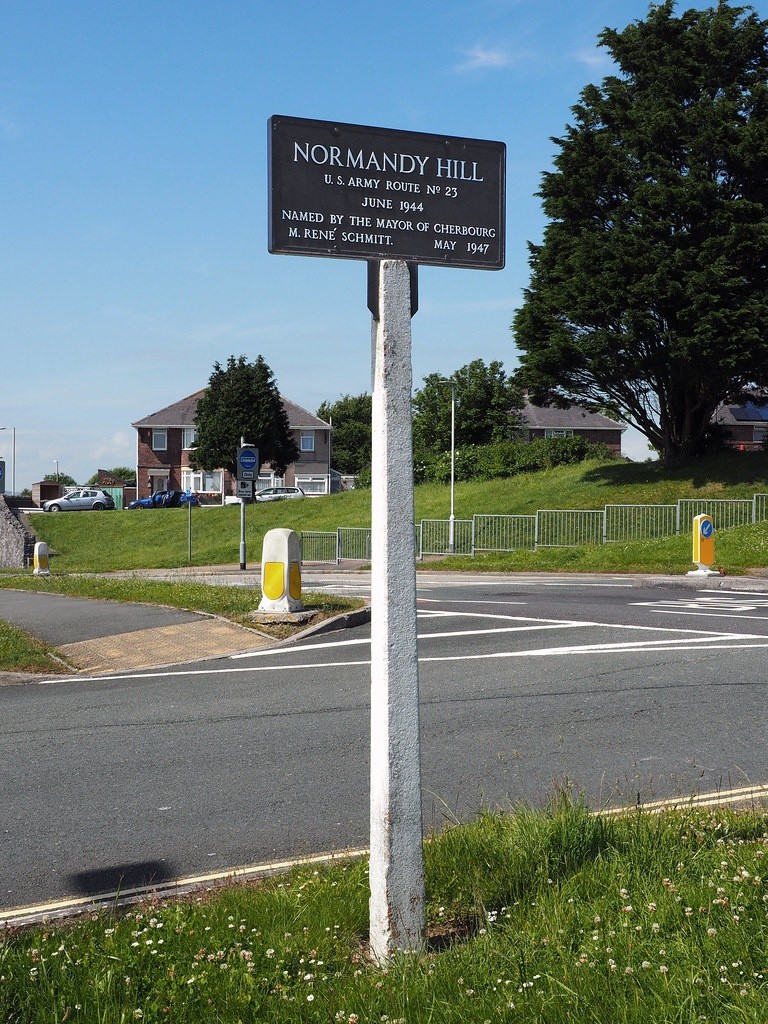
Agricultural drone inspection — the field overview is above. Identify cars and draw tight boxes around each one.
[254,487,305,503]
[43,488,114,510]
[128,489,201,509]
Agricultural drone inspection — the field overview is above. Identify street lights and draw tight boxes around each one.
[439,381,455,552]
[1,426,15,497]
[53,460,58,483]
[239,443,256,569]
